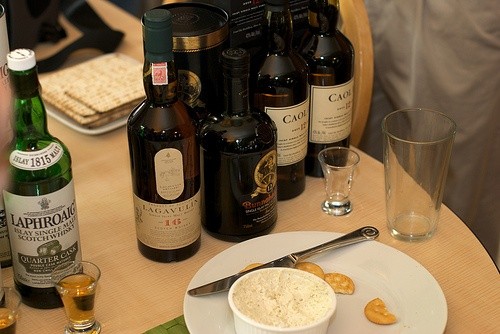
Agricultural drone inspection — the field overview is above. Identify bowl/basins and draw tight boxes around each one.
[227,267,338,334]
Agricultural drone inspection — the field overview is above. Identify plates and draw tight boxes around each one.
[183,231,448,334]
[43,104,131,135]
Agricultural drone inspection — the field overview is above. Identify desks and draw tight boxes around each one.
[0,0,500,334]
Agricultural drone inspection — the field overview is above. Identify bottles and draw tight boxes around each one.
[295,1,357,178]
[250,0,307,201]
[0,5,27,269]
[193,47,279,243]
[125,9,202,262]
[6,47,84,307]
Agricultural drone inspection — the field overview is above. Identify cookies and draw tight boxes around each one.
[364,298,397,324]
[293,262,325,281]
[323,273,355,295]
[239,262,264,273]
[38,55,147,130]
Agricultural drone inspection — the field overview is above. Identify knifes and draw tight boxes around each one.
[187,225,380,297]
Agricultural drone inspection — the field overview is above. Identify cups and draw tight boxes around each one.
[49,260,102,334]
[0,286,23,334]
[318,147,361,216]
[381,108,457,243]
